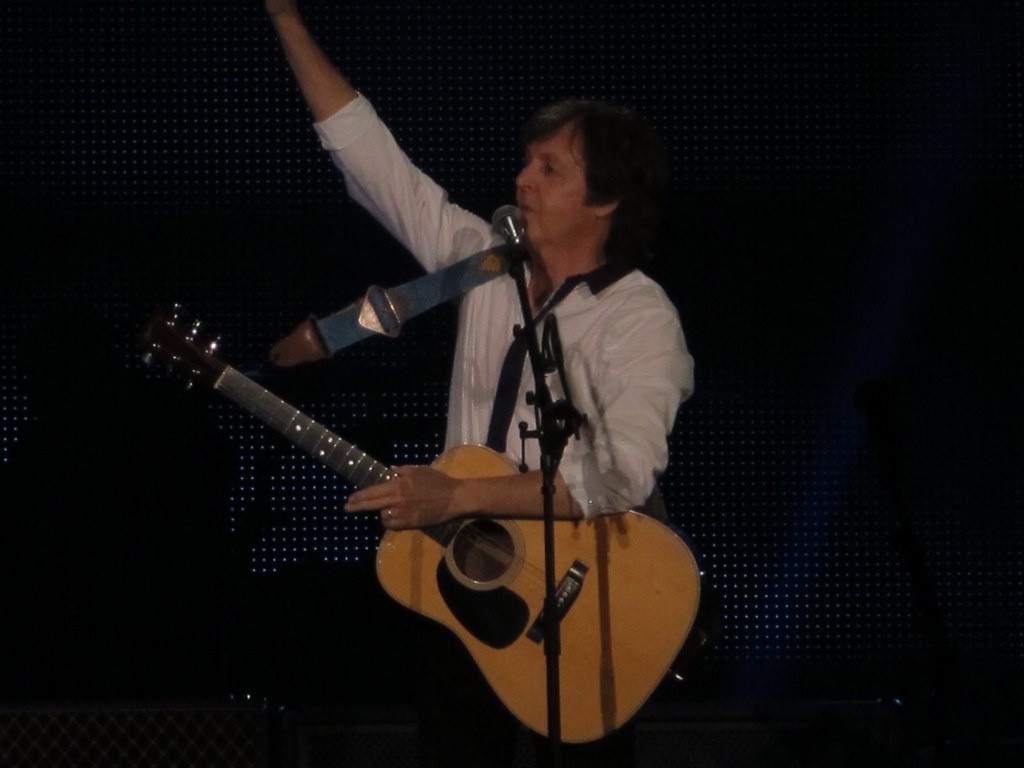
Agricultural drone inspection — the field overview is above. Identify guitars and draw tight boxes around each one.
[139,300,708,747]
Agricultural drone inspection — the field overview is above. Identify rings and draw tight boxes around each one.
[388,509,393,517]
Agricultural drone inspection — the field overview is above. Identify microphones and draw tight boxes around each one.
[492,205,523,247]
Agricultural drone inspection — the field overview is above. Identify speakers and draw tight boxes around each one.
[0,697,905,768]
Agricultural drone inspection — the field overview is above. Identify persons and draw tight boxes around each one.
[265,0,696,768]
